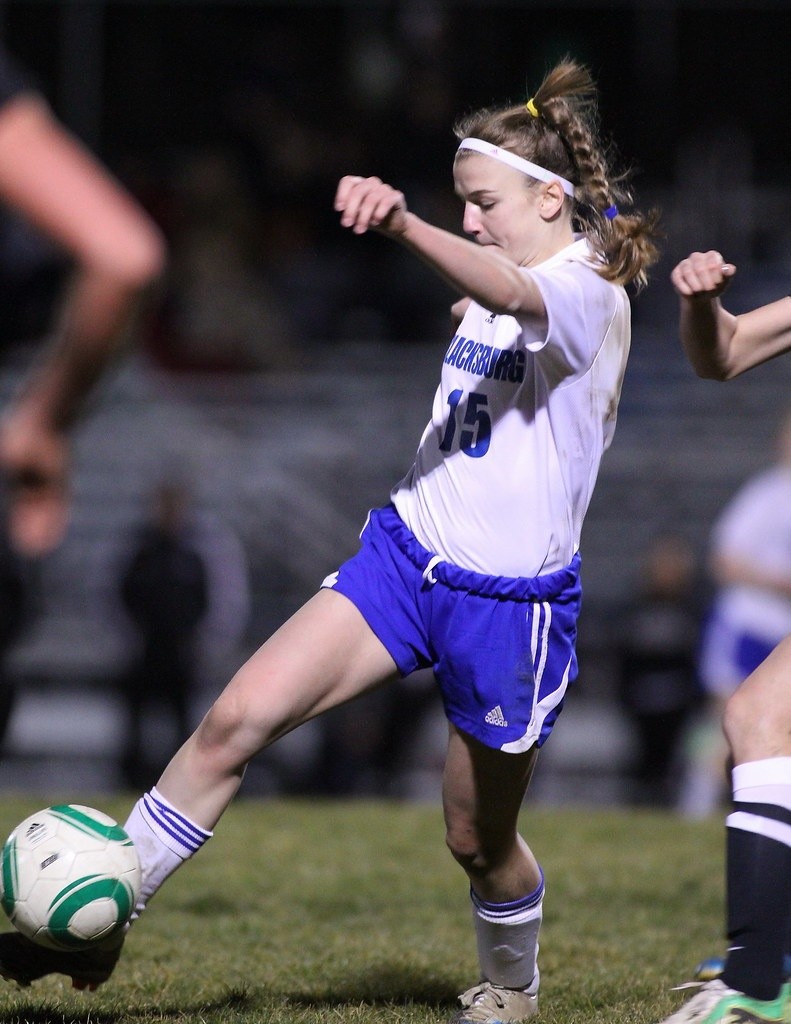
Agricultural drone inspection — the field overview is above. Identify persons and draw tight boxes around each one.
[699,413,791,810]
[0,53,659,1024]
[604,535,710,810]
[116,473,209,795]
[0,39,168,759]
[658,250,791,1024]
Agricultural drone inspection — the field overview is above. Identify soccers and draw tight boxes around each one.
[0,803,142,952]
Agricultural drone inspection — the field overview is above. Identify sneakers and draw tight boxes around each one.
[455,982,538,1024]
[0,927,126,992]
[663,979,791,1024]
